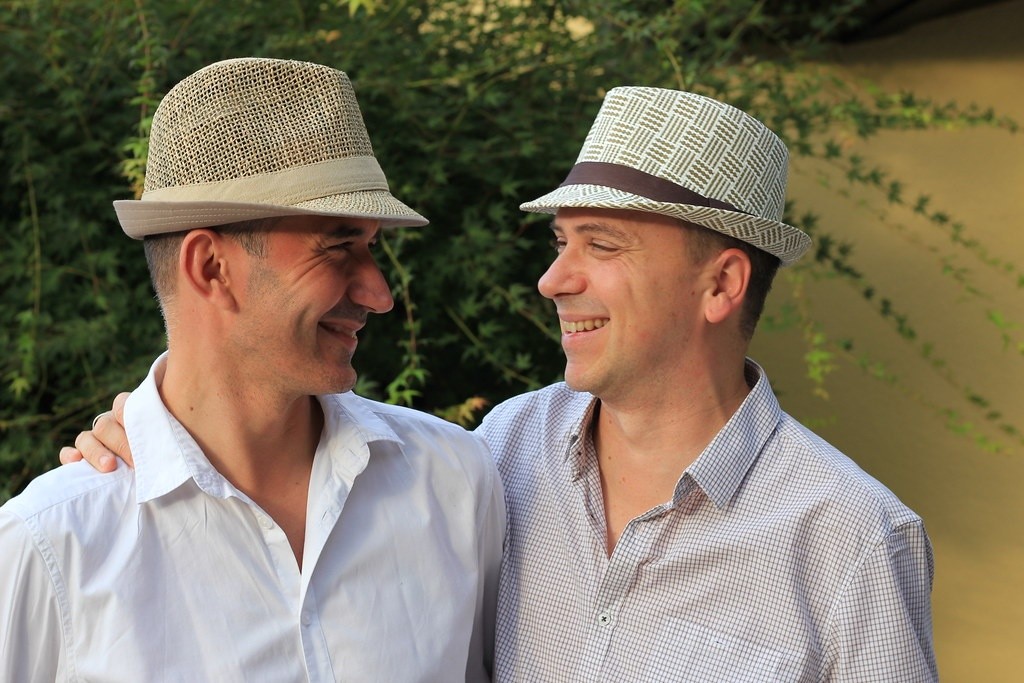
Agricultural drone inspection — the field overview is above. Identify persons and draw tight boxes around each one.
[59,87,938,683]
[0,59,507,682]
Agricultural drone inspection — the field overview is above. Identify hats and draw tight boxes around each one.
[112,58,430,239]
[520,85,813,268]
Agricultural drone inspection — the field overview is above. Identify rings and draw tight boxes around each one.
[92,411,110,430]
